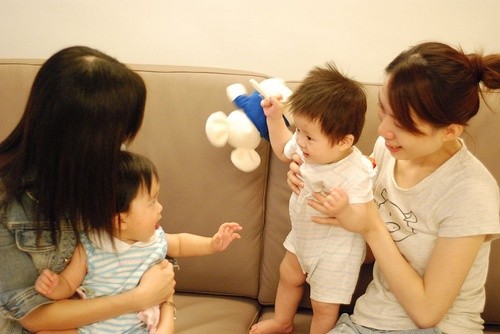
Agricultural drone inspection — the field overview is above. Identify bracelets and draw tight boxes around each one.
[165,300,181,315]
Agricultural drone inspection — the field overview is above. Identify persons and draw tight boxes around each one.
[286,40,500,333]
[245,60,375,333]
[35,149,245,334]
[1,45,175,334]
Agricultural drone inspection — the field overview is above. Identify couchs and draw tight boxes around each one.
[1,59,499,333]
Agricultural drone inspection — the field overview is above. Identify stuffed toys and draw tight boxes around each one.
[204,74,297,174]
